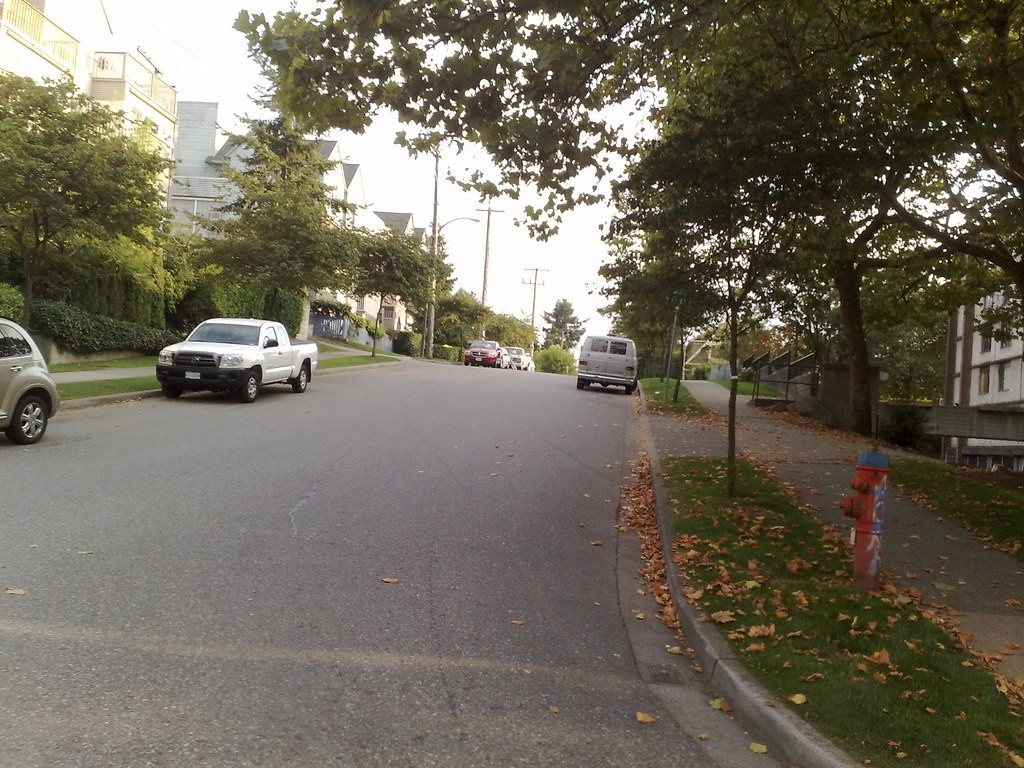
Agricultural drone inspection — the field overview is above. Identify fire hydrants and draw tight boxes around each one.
[839,439,897,596]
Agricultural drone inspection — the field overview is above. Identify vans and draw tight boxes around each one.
[576,335,643,396]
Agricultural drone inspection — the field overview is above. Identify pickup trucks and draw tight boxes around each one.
[155,318,318,403]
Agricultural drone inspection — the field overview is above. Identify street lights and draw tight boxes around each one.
[421,216,480,358]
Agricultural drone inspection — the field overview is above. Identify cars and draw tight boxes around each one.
[464,340,502,368]
[499,346,536,372]
[0,317,60,446]
